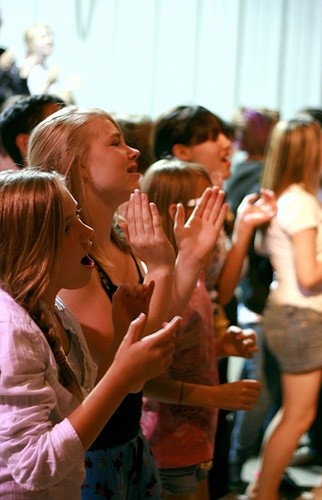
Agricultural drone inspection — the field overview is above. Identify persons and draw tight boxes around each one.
[0,20,322,496]
[139,158,264,500]
[0,167,184,500]
[27,105,228,500]
[244,111,322,500]
[153,105,278,500]
